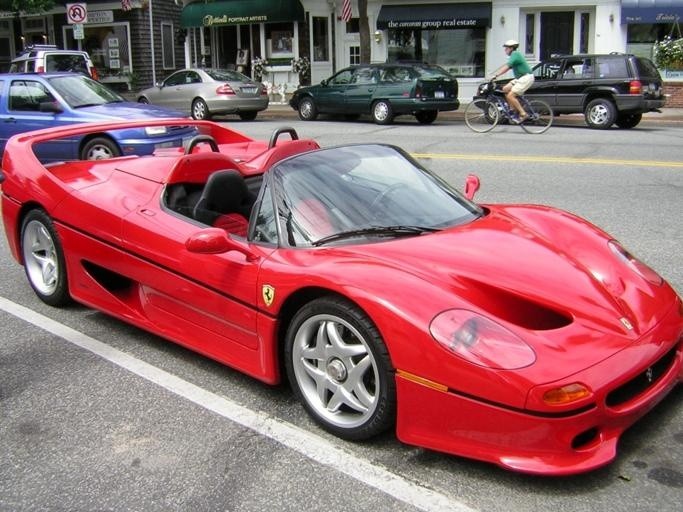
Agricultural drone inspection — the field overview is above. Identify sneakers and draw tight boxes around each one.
[514,111,531,125]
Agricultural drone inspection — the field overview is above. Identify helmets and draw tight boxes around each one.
[503,39,520,48]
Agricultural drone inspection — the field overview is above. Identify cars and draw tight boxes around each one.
[135,67,269,120]
[0,71,198,179]
[288,62,458,125]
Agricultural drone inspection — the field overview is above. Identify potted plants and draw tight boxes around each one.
[124,71,141,102]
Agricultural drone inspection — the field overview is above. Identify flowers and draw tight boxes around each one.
[290,57,310,80]
[252,56,268,78]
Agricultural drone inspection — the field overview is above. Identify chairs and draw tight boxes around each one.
[190,165,352,248]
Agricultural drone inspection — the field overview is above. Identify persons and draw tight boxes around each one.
[490,40,534,124]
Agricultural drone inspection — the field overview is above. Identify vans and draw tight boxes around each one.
[9,43,98,82]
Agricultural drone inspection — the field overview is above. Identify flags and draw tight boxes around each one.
[342,0,353,23]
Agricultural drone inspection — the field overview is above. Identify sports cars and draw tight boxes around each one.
[1,118,682,478]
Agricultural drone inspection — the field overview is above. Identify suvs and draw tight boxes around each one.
[471,52,666,128]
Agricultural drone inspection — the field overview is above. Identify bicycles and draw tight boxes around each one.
[463,76,553,134]
